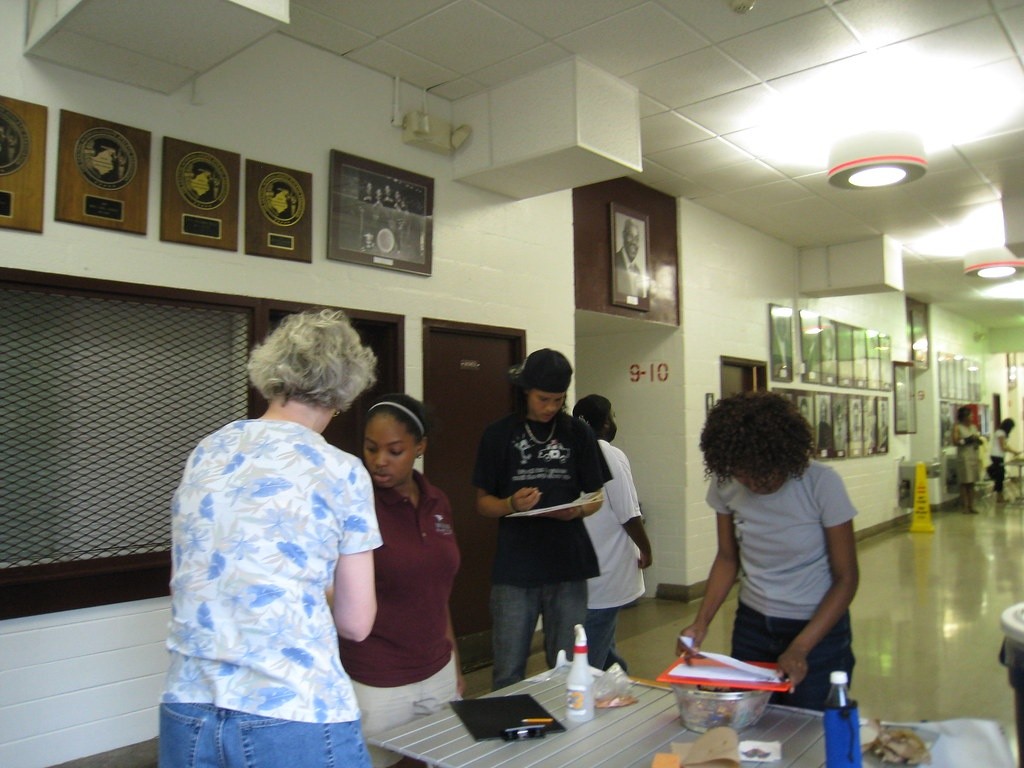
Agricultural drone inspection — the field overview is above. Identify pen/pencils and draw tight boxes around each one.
[522,719,554,722]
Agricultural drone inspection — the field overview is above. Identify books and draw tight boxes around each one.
[506,493,603,518]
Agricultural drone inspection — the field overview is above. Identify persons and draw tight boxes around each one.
[616,219,642,295]
[675,389,860,709]
[471,348,614,692]
[800,396,889,458]
[337,178,407,251]
[337,396,461,768]
[990,418,1021,503]
[952,406,985,514]
[154,309,376,768]
[573,395,654,673]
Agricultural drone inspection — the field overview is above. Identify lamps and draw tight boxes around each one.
[400,111,472,154]
[828,131,927,190]
[964,248,1024,278]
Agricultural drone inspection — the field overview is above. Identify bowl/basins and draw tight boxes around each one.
[670,683,774,733]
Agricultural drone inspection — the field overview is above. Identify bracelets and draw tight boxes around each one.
[960,440,966,445]
[507,496,517,512]
[580,506,584,516]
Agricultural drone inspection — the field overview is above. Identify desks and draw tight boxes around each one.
[999,458,1024,504]
[368,659,1014,768]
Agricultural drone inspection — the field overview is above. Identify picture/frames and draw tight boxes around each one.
[768,304,918,460]
[938,351,990,447]
[609,203,652,312]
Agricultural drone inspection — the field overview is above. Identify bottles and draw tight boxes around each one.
[821,670,863,768]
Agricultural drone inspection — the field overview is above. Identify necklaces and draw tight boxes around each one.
[525,418,556,445]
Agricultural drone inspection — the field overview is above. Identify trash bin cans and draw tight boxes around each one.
[1000,603,1024,768]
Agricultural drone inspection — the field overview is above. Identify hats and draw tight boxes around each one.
[511,349,572,393]
